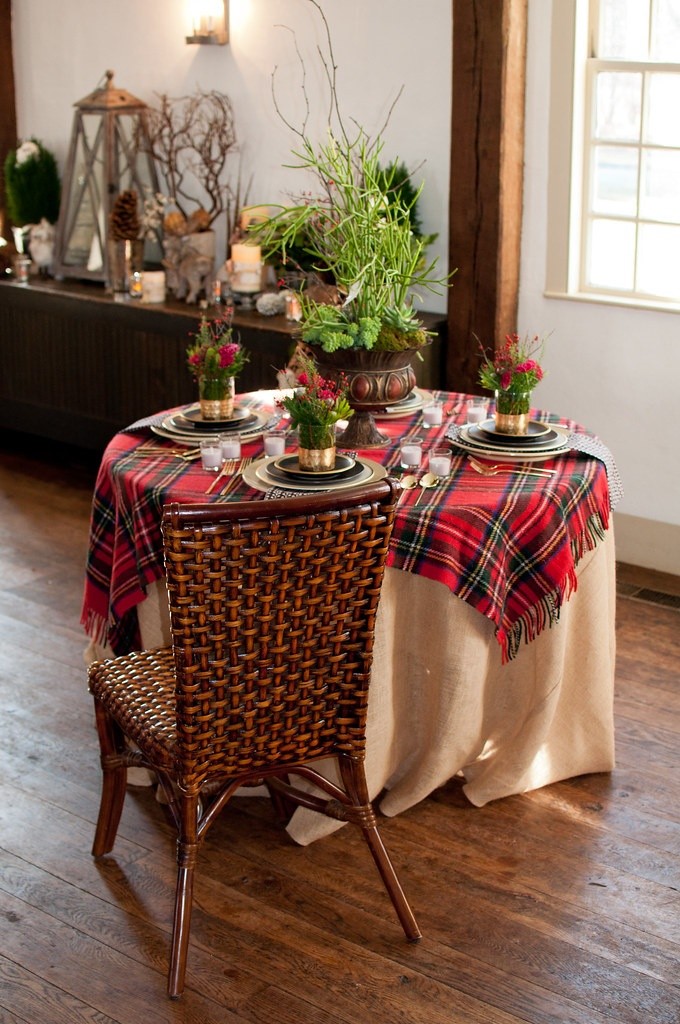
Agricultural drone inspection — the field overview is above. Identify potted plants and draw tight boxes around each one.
[5,135,62,283]
[237,1,459,406]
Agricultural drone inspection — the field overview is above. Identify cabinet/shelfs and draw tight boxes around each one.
[0,288,349,487]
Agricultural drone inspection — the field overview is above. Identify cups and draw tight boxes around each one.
[466,398,490,426]
[429,448,452,480]
[400,437,423,471]
[263,430,285,459]
[422,399,442,428]
[273,393,292,419]
[15,259,32,284]
[199,432,241,471]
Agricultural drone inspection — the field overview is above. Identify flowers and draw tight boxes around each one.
[470,327,555,415]
[270,348,355,452]
[185,295,250,401]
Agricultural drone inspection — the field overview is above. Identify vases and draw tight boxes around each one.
[298,424,336,471]
[495,390,531,435]
[199,378,236,421]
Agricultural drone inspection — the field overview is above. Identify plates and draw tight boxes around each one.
[242,453,388,492]
[370,389,435,419]
[150,406,282,446]
[443,419,570,463]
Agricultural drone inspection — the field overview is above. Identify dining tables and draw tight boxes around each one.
[80,387,615,845]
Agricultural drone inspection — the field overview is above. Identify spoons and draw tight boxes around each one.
[395,473,440,506]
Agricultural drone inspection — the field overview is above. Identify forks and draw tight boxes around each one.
[135,446,201,461]
[205,457,252,495]
[467,454,557,477]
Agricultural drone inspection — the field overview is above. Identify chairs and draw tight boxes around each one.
[87,478,424,998]
